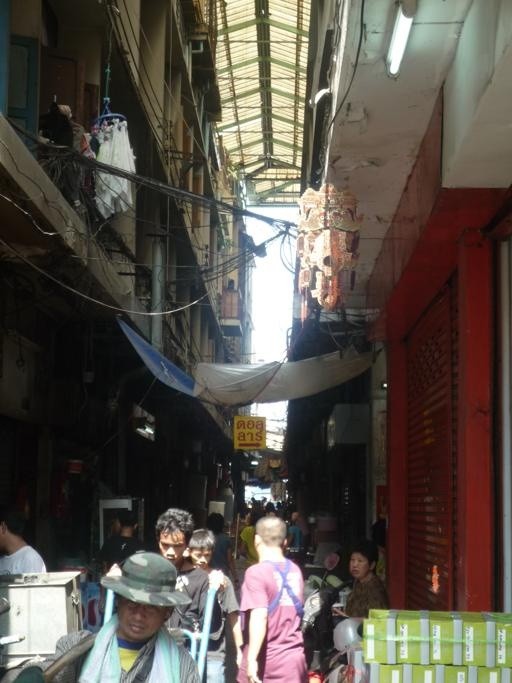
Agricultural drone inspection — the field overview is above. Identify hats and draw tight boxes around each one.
[99,552,193,607]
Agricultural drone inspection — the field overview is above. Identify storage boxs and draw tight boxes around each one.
[362,609,512,683]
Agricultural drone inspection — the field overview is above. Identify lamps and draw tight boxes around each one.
[384,0,417,79]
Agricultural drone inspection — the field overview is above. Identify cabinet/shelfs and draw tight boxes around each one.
[0,571,83,655]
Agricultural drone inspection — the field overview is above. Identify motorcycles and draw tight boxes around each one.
[301,552,368,683]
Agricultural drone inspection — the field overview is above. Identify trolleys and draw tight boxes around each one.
[101,563,221,682]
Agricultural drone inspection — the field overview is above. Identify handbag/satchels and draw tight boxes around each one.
[228,575,243,604]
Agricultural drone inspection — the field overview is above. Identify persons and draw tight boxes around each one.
[3,552,201,682]
[97,488,390,682]
[0,501,48,576]
